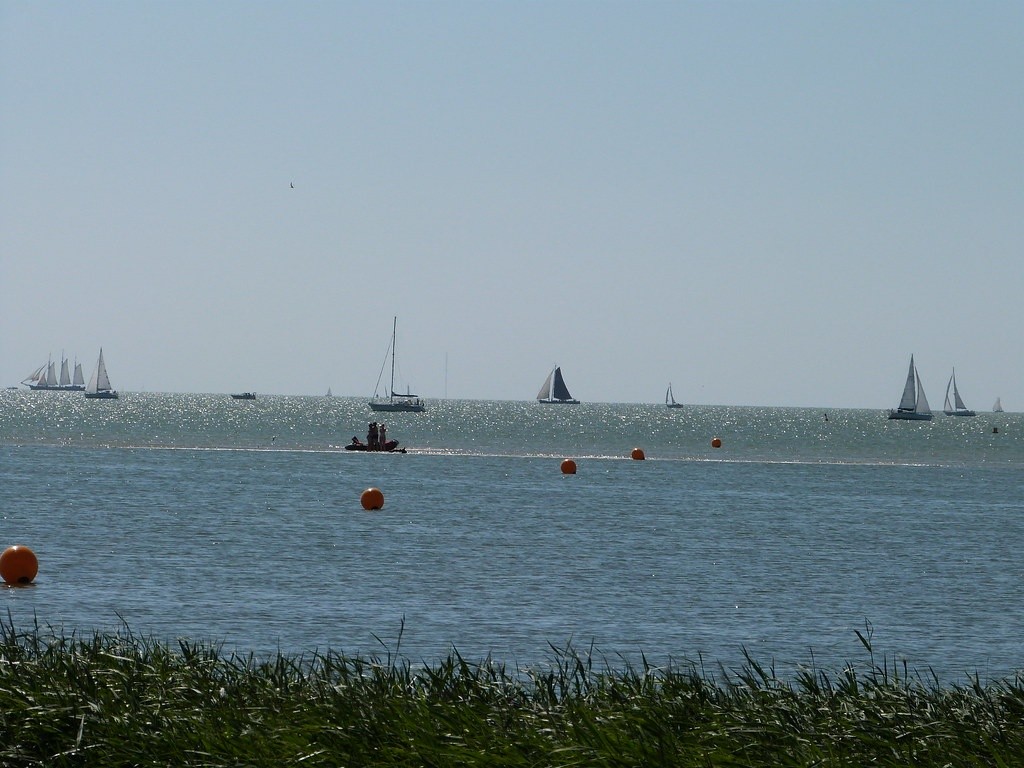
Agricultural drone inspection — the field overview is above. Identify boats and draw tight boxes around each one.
[345,438,399,451]
[231,392,256,399]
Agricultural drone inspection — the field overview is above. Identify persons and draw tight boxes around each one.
[367,422,386,452]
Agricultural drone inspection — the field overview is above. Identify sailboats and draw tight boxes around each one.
[536,365,580,404]
[943,366,976,416]
[368,316,426,413]
[992,397,1004,412]
[21,353,86,391]
[889,353,935,421]
[325,386,332,396]
[85,347,118,399]
[666,382,683,408]
